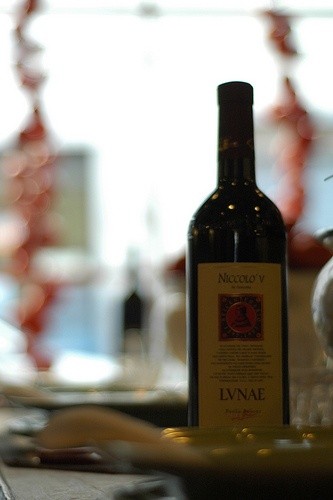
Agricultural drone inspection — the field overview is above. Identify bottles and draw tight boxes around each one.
[185,81,291,426]
